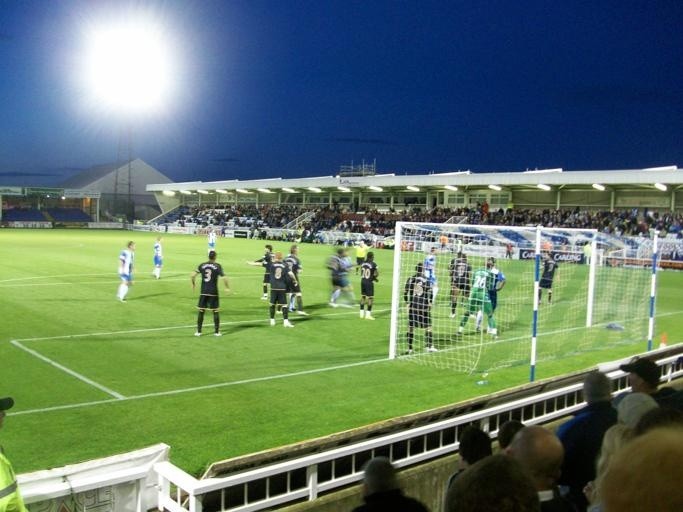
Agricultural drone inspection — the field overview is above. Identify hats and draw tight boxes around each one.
[0,397,13,411]
[620,358,660,383]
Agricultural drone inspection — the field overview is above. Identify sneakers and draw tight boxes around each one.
[297,311,309,316]
[270,318,276,325]
[365,316,375,321]
[283,323,294,328]
[215,332,221,337]
[426,348,437,352]
[194,333,201,336]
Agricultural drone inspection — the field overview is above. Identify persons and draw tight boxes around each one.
[247,245,308,327]
[448,356,682,512]
[331,242,379,320]
[404,263,436,355]
[151,239,164,279]
[350,458,430,510]
[424,248,506,335]
[116,241,136,302]
[116,200,683,270]
[538,254,559,304]
[192,251,230,334]
[0,398,30,512]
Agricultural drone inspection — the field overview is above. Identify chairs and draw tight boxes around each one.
[153,204,683,247]
